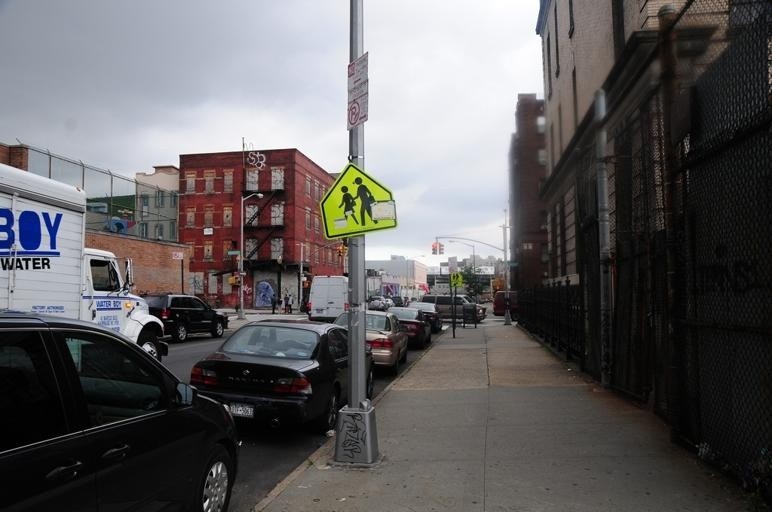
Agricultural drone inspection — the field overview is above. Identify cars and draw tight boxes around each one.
[386,306,431,349]
[330,308,409,376]
[367,290,405,312]
[190,316,376,442]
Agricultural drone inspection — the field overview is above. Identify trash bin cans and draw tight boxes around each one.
[208,296,217,308]
[463,303,477,328]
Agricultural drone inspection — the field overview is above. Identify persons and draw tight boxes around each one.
[277,296,283,314]
[284,294,288,314]
[271,294,276,314]
[288,295,293,313]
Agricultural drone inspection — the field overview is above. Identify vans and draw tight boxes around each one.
[407,301,442,333]
[422,293,486,326]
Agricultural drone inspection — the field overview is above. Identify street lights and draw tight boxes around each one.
[240,192,266,320]
[449,240,475,273]
[405,255,426,286]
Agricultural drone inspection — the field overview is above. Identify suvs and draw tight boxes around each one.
[143,289,230,342]
[1,306,245,511]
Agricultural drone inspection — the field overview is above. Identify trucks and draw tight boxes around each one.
[306,272,350,324]
[0,162,171,380]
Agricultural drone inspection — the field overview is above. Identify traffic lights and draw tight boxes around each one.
[431,243,438,255]
[336,244,343,257]
[342,245,348,256]
[441,245,445,254]
